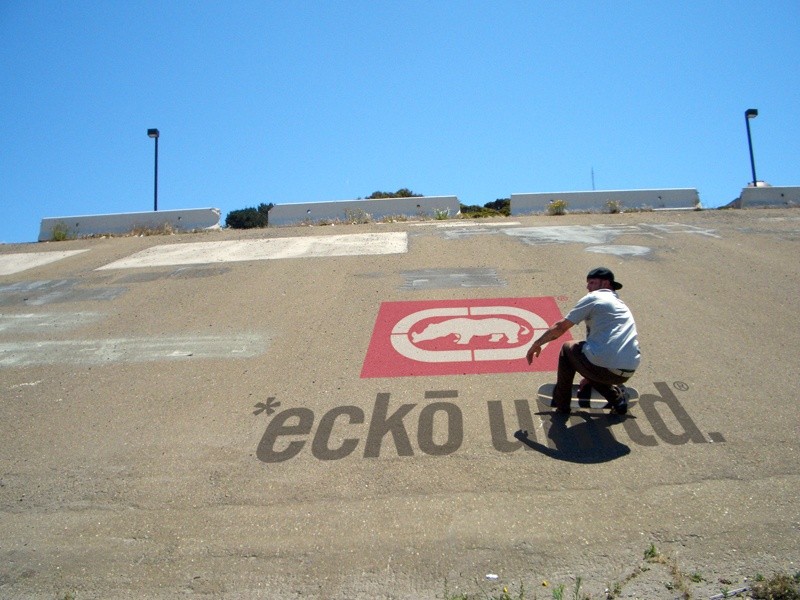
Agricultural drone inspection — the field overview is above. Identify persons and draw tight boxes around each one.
[526,267,641,415]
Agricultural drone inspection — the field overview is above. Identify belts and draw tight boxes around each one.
[608,367,635,377]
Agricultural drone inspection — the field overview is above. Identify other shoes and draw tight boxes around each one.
[611,402,628,416]
[554,406,572,421]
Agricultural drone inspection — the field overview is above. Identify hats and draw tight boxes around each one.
[586,267,623,290]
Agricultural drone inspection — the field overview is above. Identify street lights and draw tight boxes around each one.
[744,108,759,186]
[146,128,160,211]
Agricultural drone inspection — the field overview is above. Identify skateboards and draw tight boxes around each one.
[537,383,641,410]
